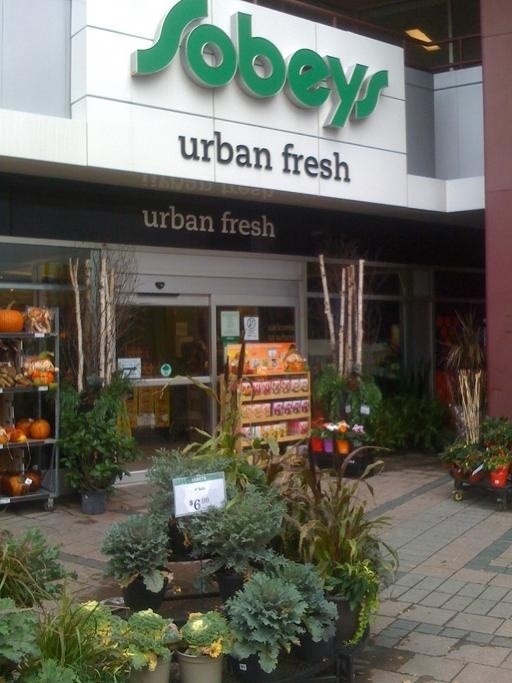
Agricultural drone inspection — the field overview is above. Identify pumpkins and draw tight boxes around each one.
[17,419,34,436]
[25,473,41,492]
[1,301,23,333]
[4,476,26,496]
[32,367,53,385]
[31,419,51,439]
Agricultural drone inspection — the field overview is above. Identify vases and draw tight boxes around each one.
[310,436,350,454]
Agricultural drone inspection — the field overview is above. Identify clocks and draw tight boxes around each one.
[158,361,174,378]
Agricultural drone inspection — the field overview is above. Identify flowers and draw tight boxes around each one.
[311,415,374,447]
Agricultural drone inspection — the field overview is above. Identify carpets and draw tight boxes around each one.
[112,468,155,490]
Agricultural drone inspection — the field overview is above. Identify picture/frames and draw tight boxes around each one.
[242,315,261,343]
[221,311,240,340]
[117,357,142,379]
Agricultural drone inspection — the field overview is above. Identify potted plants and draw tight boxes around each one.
[99,445,401,681]
[475,415,512,448]
[483,449,512,489]
[59,367,144,514]
[441,443,488,481]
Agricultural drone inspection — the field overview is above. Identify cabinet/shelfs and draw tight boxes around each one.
[0,307,59,511]
[126,387,138,429]
[186,383,210,451]
[220,355,311,466]
[138,361,170,427]
[219,373,241,434]
